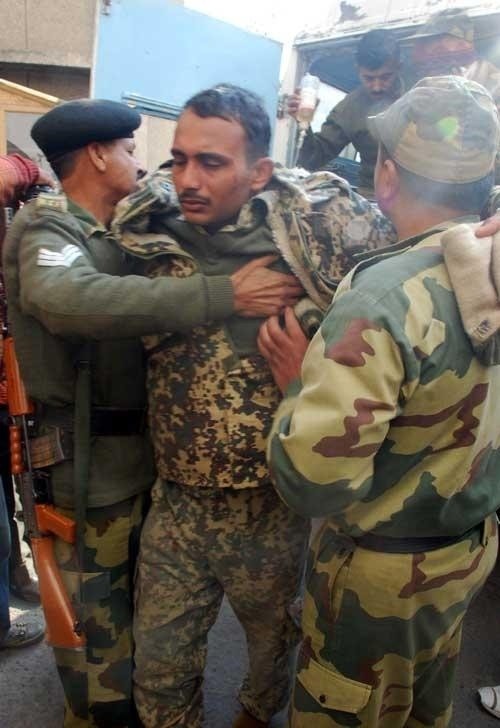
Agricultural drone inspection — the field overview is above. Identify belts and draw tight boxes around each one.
[45,405,150,436]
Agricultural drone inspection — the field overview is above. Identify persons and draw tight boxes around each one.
[287,29,410,201]
[1,99,306,726]
[257,76,500,727]
[0,153,52,647]
[399,8,497,99]
[108,83,500,727]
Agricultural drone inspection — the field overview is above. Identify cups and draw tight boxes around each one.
[295,75,322,121]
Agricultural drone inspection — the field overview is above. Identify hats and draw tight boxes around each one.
[367,75,499,186]
[395,7,474,45]
[31,98,142,163]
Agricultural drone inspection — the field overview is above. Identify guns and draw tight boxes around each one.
[0,205,89,649]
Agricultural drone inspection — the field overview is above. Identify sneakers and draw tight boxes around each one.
[9,561,42,602]
[477,685,500,719]
[0,620,45,650]
